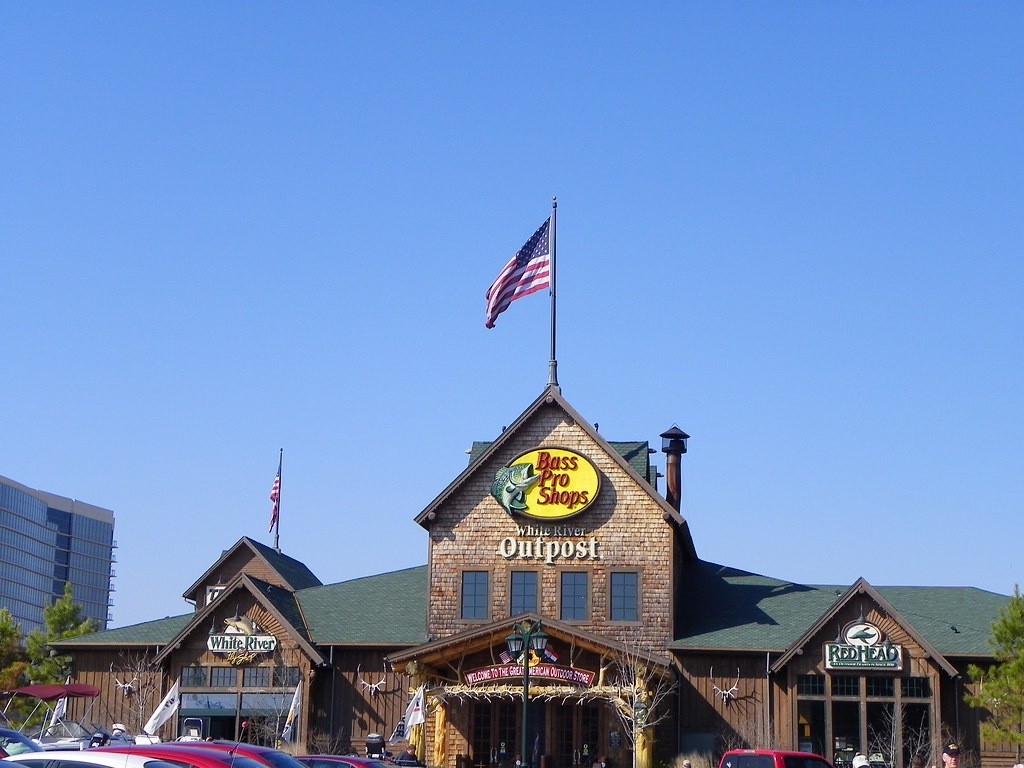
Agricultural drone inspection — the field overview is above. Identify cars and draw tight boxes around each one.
[0,683,400,768]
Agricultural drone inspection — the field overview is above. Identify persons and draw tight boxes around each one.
[682,760,692,768]
[852,754,871,768]
[346,746,360,757]
[394,745,419,767]
[942,743,961,768]
[911,753,927,768]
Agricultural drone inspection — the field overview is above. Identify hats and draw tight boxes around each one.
[942,743,960,755]
[852,755,870,768]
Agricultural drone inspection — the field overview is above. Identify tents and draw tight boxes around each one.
[0,683,129,746]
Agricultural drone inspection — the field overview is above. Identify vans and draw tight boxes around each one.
[718,748,834,768]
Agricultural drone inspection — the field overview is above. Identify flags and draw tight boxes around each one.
[143,676,180,736]
[388,684,425,746]
[483,216,550,329]
[281,680,302,745]
[268,453,280,533]
[45,677,69,736]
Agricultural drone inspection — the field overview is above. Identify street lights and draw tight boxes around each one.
[504,619,553,768]
